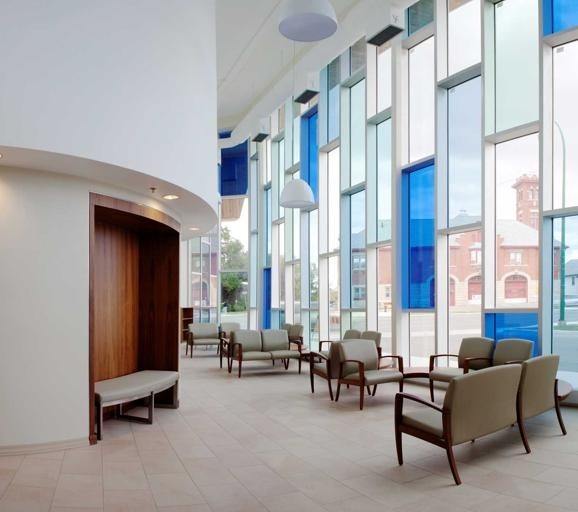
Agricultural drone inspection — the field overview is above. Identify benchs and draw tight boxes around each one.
[93,367,181,441]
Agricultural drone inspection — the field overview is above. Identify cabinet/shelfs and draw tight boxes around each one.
[180,306,195,344]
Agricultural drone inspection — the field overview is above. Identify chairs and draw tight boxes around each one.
[395,363,532,486]
[187,321,316,379]
[309,321,574,411]
[469,354,567,454]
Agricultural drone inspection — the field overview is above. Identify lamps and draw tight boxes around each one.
[278,40,317,215]
[277,1,340,43]
